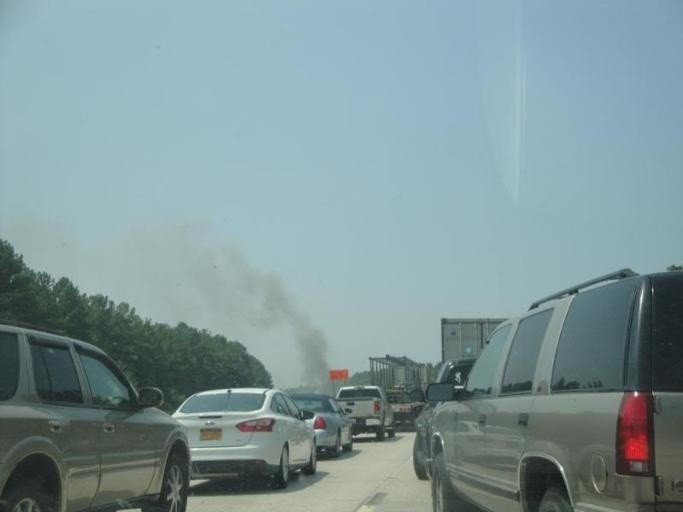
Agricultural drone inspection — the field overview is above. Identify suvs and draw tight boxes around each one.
[422,265,682,511]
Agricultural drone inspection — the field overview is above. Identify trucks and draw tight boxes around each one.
[369,355,422,398]
[441,318,510,363]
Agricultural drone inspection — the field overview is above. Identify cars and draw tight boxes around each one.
[0,323,191,511]
[170,387,318,489]
[286,393,354,457]
[413,357,478,481]
[383,387,424,428]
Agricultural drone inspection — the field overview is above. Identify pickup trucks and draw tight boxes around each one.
[332,384,396,441]
